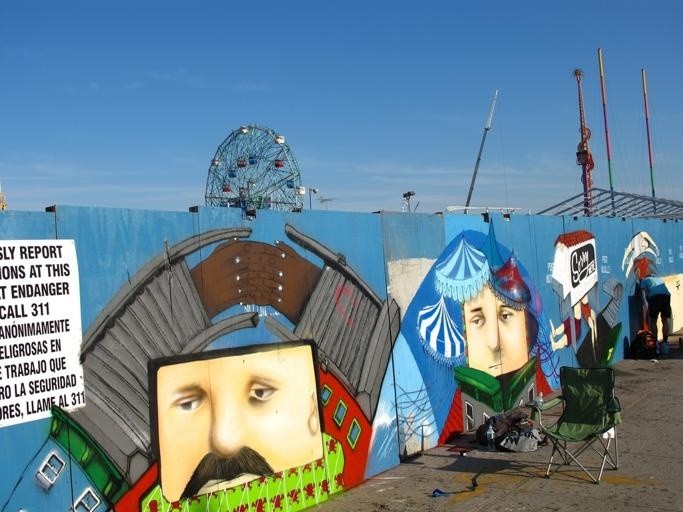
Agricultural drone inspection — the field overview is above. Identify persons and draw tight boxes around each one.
[463,282,528,379]
[155,344,324,503]
[641,274,673,352]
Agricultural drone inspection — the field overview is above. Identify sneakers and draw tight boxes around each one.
[655,342,669,354]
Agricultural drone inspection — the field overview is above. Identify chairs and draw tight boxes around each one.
[526,366,620,485]
